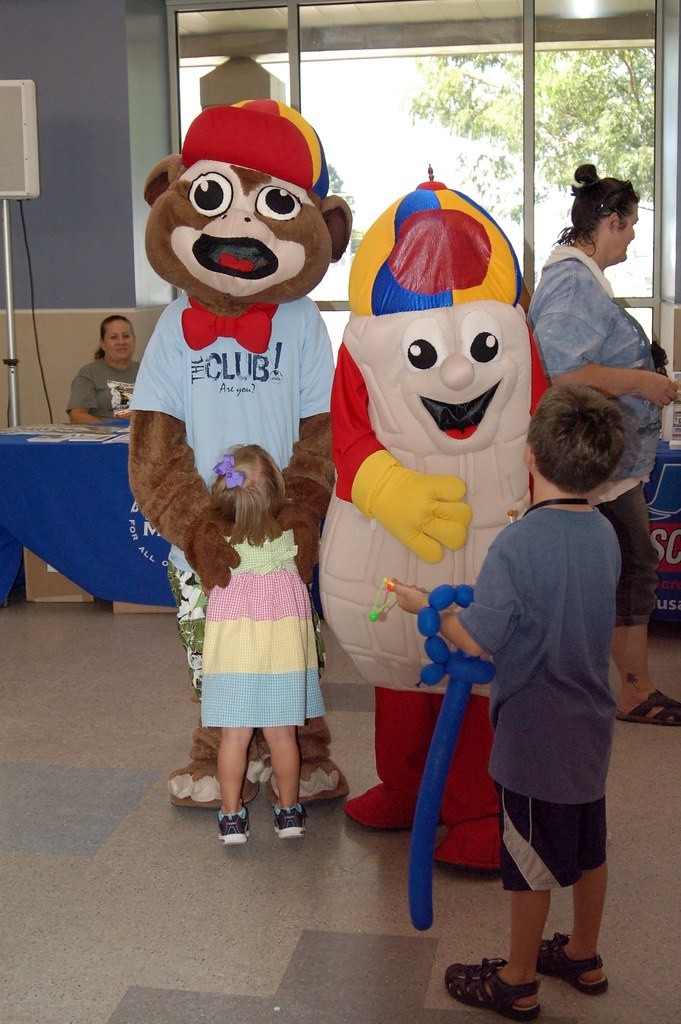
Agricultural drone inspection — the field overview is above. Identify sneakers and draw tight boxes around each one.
[272,801,306,839]
[217,799,249,843]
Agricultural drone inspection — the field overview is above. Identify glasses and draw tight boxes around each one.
[596,180,633,212]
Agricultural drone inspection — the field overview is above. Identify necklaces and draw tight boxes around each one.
[522,498,589,518]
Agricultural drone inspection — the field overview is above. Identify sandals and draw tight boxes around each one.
[445,958,540,1021]
[534,932,608,995]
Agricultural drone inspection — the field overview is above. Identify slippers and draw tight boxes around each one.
[615,689,681,726]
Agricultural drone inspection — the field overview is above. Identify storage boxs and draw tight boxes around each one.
[22,546,181,614]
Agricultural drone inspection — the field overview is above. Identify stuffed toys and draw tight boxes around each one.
[128,99,351,808]
[319,181,546,871]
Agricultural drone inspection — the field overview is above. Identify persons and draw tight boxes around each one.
[395,386,627,1023]
[528,163,681,728]
[67,314,140,424]
[200,444,329,844]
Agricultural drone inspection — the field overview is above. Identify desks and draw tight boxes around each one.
[0,419,178,615]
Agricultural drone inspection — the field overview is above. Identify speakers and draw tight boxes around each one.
[0,77,40,199]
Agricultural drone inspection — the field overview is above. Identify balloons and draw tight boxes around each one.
[409,585,495,931]
